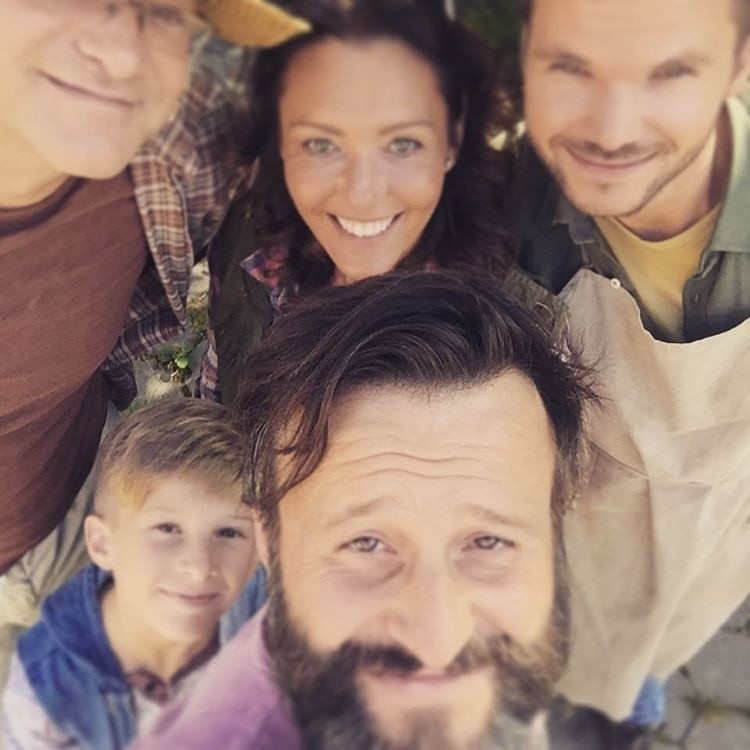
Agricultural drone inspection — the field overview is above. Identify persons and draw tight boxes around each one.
[0,397,263,750]
[198,0,530,413]
[117,267,616,750]
[0,0,312,694]
[513,0,750,728]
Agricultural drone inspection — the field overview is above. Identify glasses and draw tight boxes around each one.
[87,0,212,61]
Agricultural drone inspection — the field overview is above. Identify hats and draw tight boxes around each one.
[200,0,313,48]
